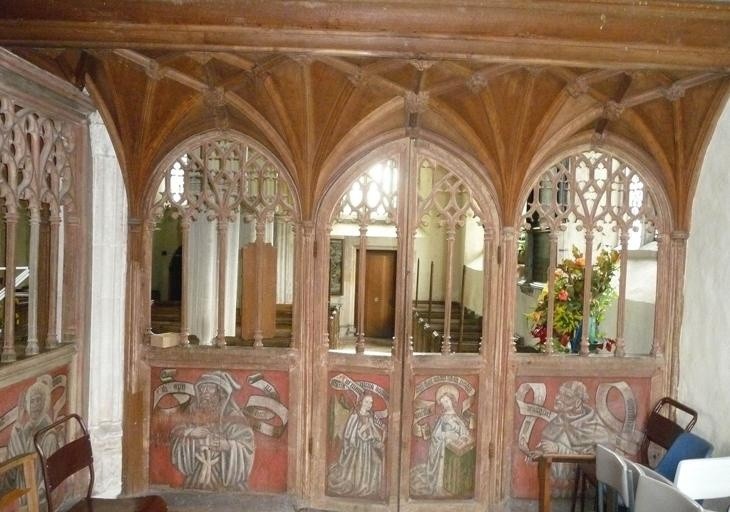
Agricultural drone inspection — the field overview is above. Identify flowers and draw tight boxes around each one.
[519,243,621,354]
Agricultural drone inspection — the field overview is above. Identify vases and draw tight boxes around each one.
[571,314,599,352]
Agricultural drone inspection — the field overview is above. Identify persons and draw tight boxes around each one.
[171,371,255,490]
[411,385,476,500]
[521,381,611,497]
[327,393,386,501]
[4,378,64,511]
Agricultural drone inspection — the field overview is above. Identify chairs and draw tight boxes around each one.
[565,398,730,512]
[34,413,167,512]
[0,451,39,512]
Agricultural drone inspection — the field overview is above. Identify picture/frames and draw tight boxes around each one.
[328,239,345,296]
